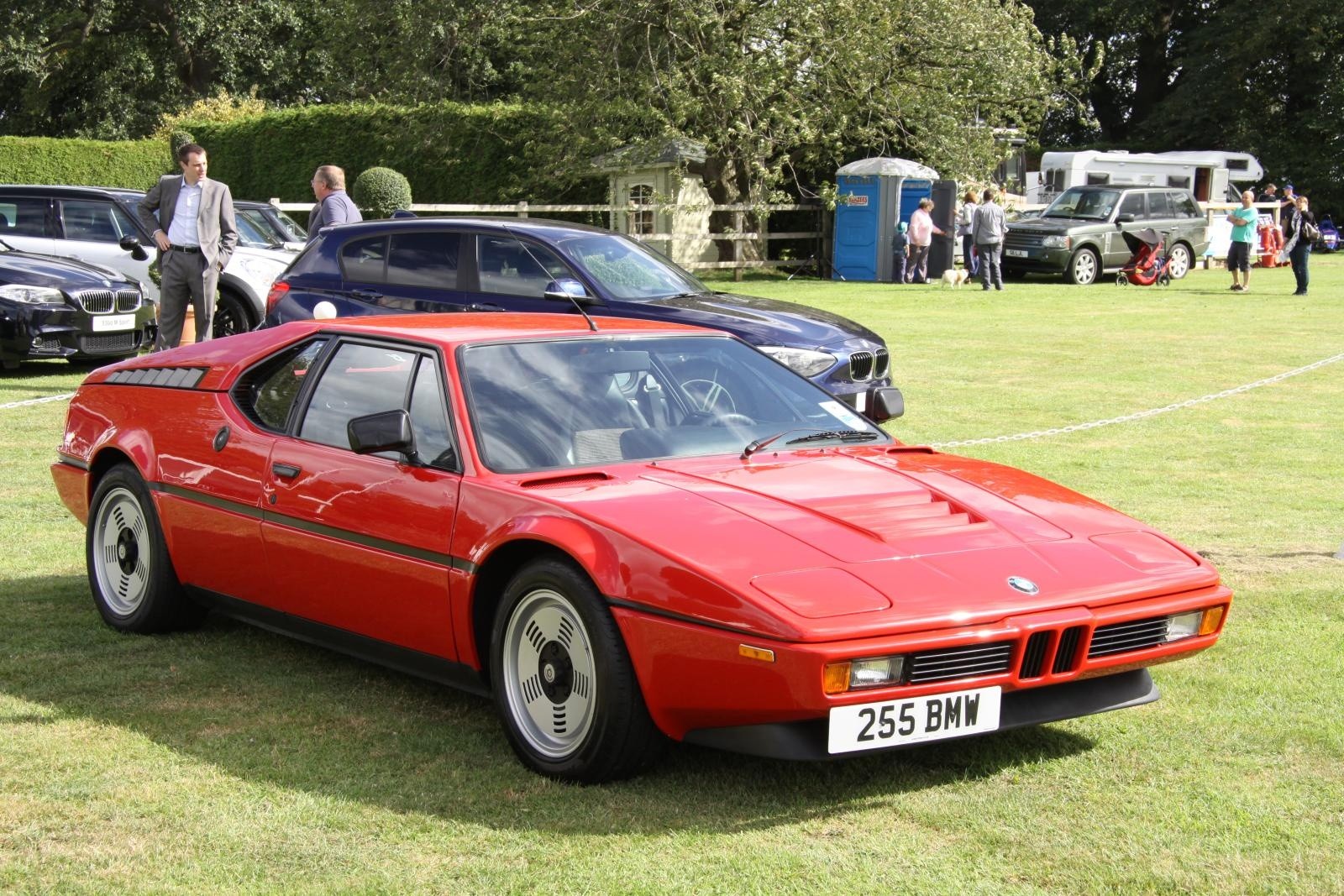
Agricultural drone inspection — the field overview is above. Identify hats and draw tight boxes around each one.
[896,221,909,232]
[1283,184,1293,190]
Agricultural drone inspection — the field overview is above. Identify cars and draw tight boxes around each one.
[0,237,159,378]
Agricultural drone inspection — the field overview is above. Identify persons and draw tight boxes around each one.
[905,197,945,283]
[137,144,238,353]
[952,190,981,277]
[891,221,910,284]
[1284,196,1316,294]
[306,165,362,245]
[1257,183,1276,213]
[1227,190,1258,291]
[1280,185,1300,233]
[970,189,1006,289]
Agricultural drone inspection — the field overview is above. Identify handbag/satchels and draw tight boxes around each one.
[1302,219,1320,243]
[969,244,981,276]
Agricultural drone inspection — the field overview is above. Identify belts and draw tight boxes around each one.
[169,245,201,253]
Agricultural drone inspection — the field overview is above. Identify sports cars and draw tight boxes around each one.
[47,308,1232,787]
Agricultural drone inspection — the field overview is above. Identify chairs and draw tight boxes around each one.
[479,239,554,304]
[532,359,648,458]
[69,211,94,239]
[0,212,18,234]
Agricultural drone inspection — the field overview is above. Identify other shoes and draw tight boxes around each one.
[1292,288,1307,295]
[1230,283,1242,291]
[964,277,971,284]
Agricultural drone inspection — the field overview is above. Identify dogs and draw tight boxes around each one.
[940,269,968,289]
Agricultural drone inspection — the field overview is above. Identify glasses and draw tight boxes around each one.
[310,179,322,188]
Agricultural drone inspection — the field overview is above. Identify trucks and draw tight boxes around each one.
[1036,150,1265,269]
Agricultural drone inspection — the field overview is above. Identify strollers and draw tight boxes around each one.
[1115,227,1175,287]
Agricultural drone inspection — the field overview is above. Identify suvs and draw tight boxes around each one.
[0,182,308,371]
[1001,183,1213,285]
[254,209,907,431]
